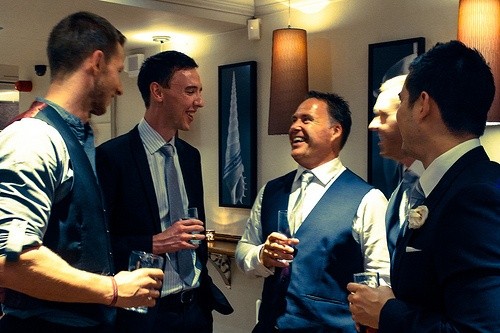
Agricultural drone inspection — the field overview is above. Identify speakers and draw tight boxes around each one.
[123,53,144,77]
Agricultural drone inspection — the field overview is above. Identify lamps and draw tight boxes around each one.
[269,0,309,136]
[457,0,500,126]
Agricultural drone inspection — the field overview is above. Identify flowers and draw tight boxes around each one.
[404,206,429,229]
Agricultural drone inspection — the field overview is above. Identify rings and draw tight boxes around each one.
[193,235,195,240]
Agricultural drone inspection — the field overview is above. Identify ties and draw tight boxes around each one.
[403,183,424,235]
[398,169,417,228]
[289,172,315,235]
[160,142,195,287]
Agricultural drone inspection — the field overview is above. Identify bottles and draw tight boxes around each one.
[204,229,215,241]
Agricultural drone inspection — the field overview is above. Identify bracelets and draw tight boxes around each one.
[107,278,118,307]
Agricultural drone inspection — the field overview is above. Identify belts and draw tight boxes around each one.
[158,287,203,310]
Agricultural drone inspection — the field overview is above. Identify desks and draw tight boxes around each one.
[206,240,237,290]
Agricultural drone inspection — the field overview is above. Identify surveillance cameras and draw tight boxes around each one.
[35,65,46,76]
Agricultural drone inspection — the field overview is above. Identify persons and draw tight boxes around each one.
[95,50,234,332]
[368,74,425,257]
[0,12,164,332]
[347,41,500,332]
[236,91,391,333]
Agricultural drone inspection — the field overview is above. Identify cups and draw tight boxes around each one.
[353,273,380,289]
[121,250,165,313]
[177,207,201,246]
[276,208,296,263]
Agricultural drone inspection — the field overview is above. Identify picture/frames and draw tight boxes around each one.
[218,61,258,209]
[368,36,425,201]
